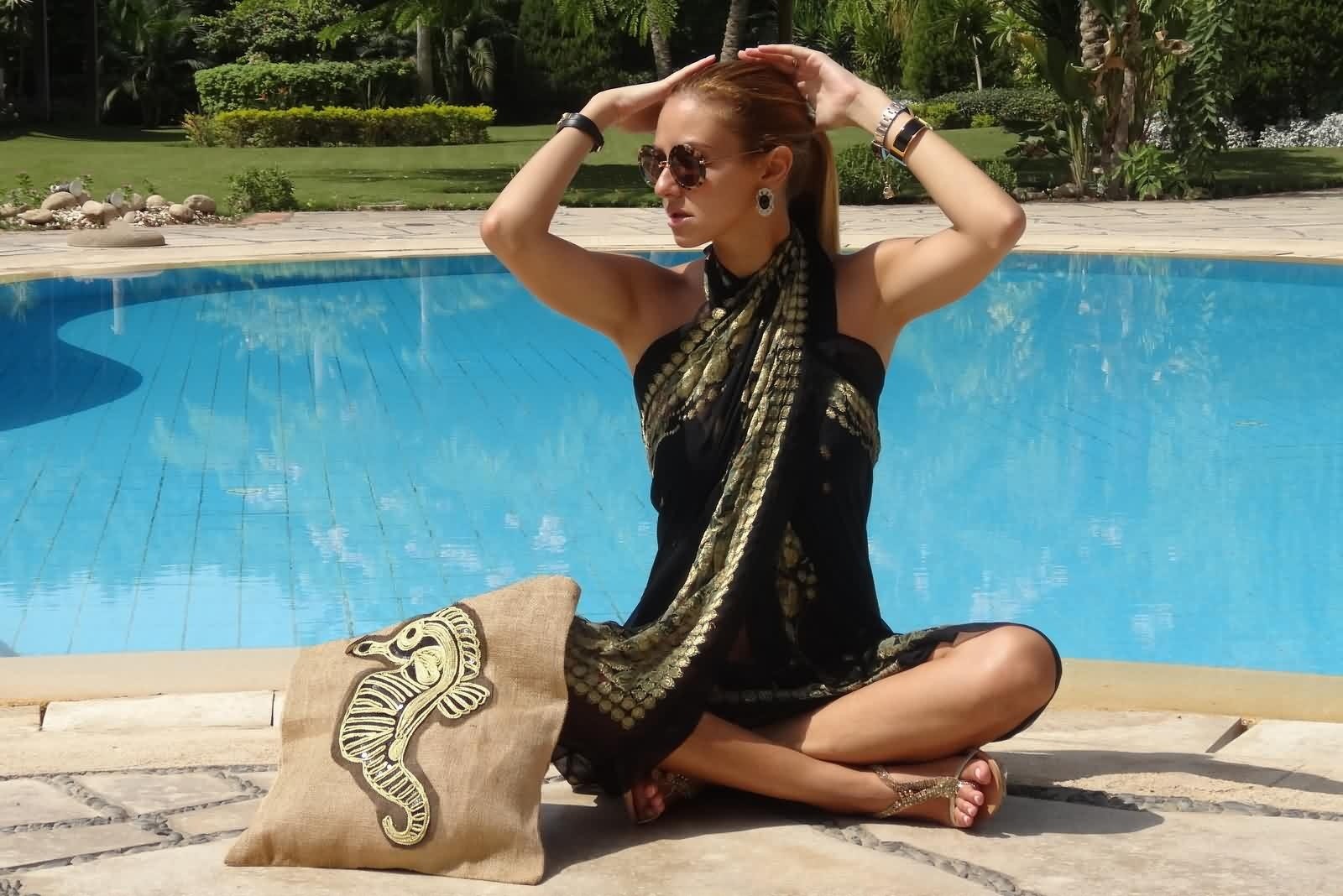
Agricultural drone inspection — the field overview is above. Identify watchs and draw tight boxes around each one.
[554,111,605,154]
[868,99,914,159]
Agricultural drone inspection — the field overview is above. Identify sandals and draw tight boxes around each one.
[863,746,1006,830]
[624,772,696,826]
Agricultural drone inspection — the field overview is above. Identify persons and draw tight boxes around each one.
[479,45,1064,829]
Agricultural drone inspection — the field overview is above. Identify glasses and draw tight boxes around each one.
[637,142,774,190]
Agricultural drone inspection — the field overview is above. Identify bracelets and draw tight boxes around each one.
[884,114,934,168]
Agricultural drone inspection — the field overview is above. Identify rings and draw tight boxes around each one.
[792,57,800,67]
